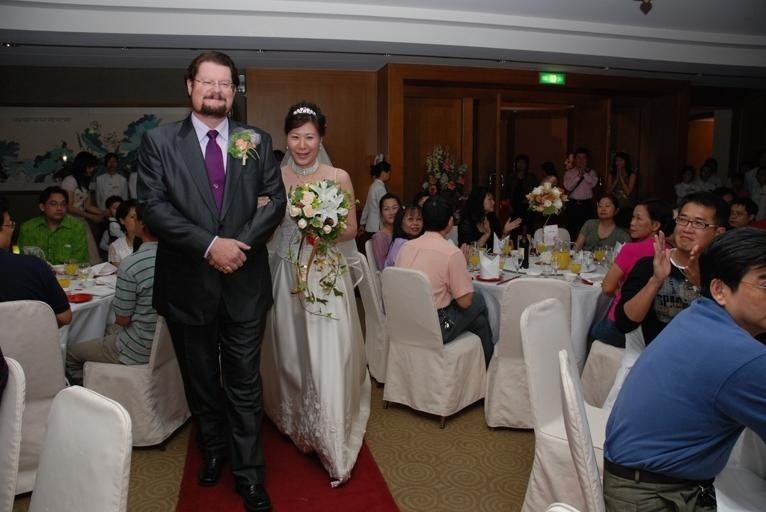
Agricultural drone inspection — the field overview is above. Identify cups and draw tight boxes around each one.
[57,257,92,293]
[470,242,613,283]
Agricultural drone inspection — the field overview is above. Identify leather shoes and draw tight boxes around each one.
[197,456,226,487]
[235,482,271,512]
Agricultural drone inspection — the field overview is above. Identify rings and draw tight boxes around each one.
[225,265,230,270]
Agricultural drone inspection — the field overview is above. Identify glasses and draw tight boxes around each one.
[193,78,235,88]
[673,216,720,229]
[1,221,16,231]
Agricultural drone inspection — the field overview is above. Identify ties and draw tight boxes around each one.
[205,129,226,216]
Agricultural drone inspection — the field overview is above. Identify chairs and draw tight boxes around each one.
[337,226,572,432]
[83,313,190,450]
[26,385,133,510]
[521,215,765,511]
[1,356,25,511]
[1,299,71,501]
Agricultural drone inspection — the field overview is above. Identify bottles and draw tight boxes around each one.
[517,226,529,268]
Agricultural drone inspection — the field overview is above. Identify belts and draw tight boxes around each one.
[603,456,688,484]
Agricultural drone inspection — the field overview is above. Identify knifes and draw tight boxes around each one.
[495,274,522,286]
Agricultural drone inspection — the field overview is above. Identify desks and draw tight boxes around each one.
[48,264,116,384]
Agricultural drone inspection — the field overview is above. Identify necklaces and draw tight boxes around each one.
[290,158,321,176]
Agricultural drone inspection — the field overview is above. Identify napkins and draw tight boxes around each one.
[80,262,118,279]
[88,275,118,289]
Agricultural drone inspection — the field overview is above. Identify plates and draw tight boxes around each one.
[67,294,92,303]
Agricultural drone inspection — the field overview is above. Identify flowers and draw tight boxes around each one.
[281,180,362,320]
[227,127,263,165]
[421,147,469,195]
[528,172,570,213]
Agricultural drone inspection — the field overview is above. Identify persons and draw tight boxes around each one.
[134,52,286,512]
[254,102,373,489]
[0,153,159,390]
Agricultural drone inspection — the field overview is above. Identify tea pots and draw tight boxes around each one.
[552,237,577,269]
